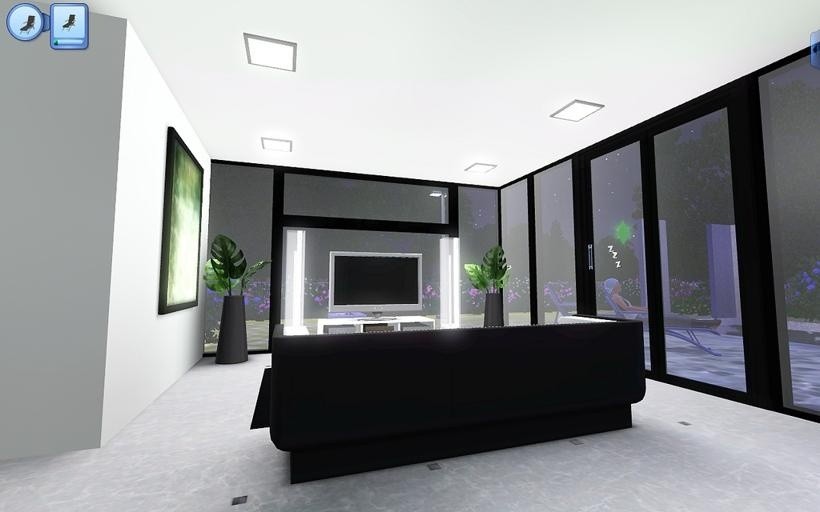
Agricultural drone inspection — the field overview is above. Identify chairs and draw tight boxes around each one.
[547,288,578,322]
[603,290,725,358]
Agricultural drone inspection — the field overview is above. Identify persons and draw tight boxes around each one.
[603,275,723,330]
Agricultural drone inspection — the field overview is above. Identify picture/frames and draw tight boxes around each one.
[158,125,205,314]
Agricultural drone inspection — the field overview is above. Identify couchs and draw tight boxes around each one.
[268,319,646,484]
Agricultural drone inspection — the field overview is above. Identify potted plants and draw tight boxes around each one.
[202,233,272,364]
[465,242,513,327]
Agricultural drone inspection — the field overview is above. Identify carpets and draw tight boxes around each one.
[249,367,270,429]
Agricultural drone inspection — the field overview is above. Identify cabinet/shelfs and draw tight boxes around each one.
[316,315,436,335]
